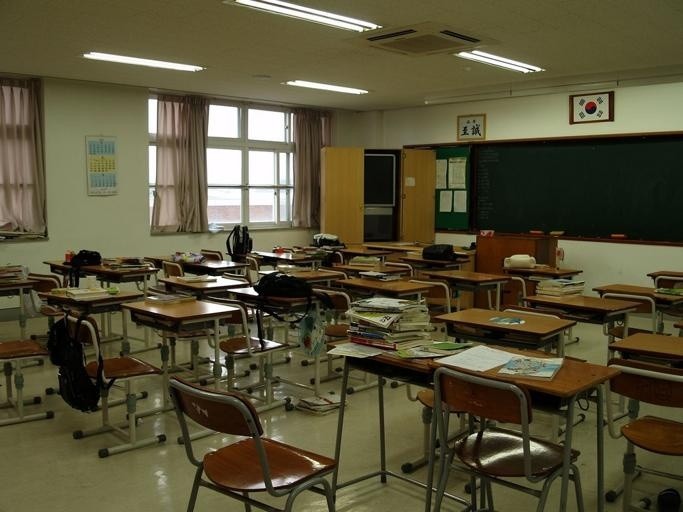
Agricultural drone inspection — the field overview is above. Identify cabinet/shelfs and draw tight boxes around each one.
[320,146,437,243]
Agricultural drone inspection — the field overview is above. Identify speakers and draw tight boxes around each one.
[364,207,393,242]
[364,154,396,207]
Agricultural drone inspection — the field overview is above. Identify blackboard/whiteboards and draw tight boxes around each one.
[475,131,683,246]
[403,142,477,234]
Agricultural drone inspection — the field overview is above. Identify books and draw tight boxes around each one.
[535,278,585,296]
[1,264,30,283]
[145,293,197,303]
[343,297,434,351]
[497,354,564,382]
[65,289,109,297]
[652,287,682,297]
[359,271,401,276]
[323,245,344,249]
[177,274,216,283]
[361,275,400,281]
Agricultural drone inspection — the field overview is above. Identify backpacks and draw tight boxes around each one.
[254,271,312,298]
[63,249,101,266]
[46,310,115,412]
[226,224,253,256]
[423,245,456,261]
[313,233,339,244]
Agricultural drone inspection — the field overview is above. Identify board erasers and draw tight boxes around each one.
[529,231,544,235]
[549,231,564,236]
[609,234,625,238]
